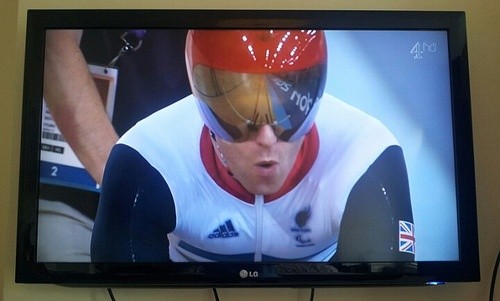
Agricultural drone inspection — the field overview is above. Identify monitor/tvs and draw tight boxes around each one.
[15,9,481,286]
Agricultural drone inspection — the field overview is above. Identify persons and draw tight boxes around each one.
[87,28,416,262]
[43,28,120,189]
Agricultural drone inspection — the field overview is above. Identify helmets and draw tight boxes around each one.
[184,29,328,142]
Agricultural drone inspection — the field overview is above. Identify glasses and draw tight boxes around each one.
[191,64,326,141]
